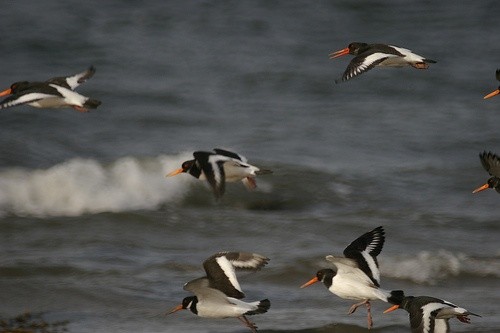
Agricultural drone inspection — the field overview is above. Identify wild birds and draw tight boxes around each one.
[328,41,439,83]
[167,250,271,333]
[165,148,274,200]
[472,149,500,195]
[302,223,405,330]
[382,295,483,333]
[0,66,101,113]
